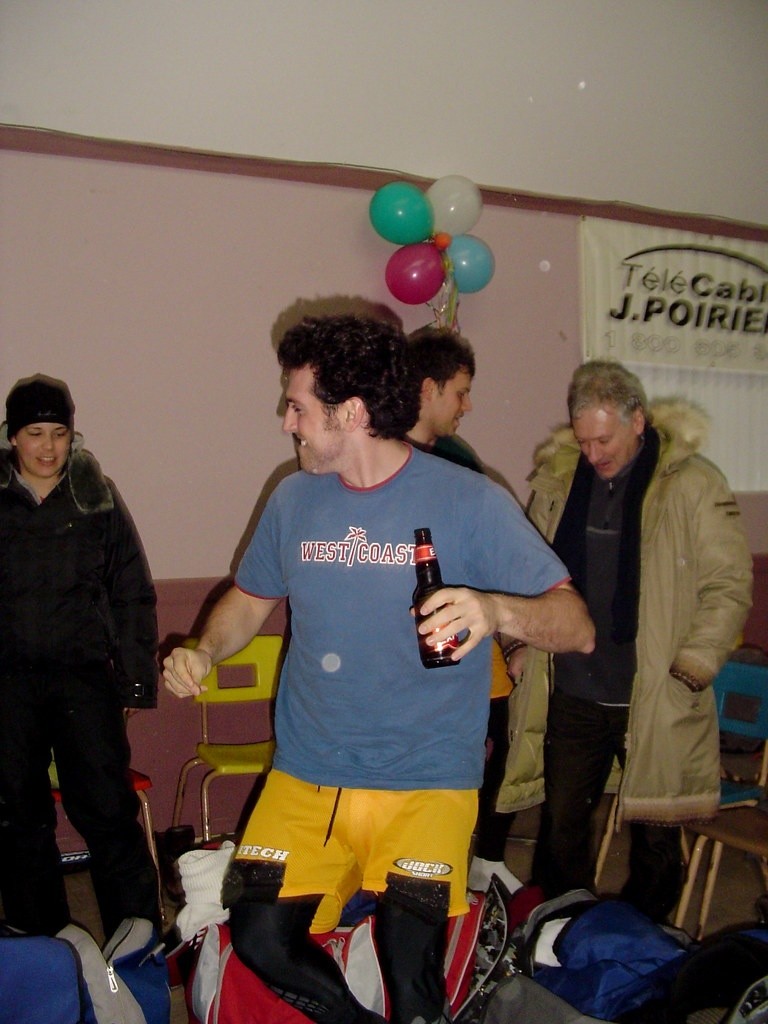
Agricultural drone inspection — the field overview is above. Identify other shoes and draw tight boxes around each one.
[465,854,523,898]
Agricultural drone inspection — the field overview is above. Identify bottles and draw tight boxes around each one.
[411,527,461,668]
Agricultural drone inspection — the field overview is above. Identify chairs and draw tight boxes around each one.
[589,656,768,941]
[43,709,167,922]
[171,635,288,841]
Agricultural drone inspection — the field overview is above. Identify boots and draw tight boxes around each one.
[154,825,195,902]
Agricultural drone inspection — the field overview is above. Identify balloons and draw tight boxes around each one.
[370,181,434,245]
[385,243,445,305]
[446,233,495,293]
[425,175,483,238]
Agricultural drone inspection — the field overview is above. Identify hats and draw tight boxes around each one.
[6,373,69,442]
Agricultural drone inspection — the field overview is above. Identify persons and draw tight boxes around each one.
[495,363,753,923]
[163,312,596,1024]
[0,374,158,942]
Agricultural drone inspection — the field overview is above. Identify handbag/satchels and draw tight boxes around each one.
[478,889,695,1024]
[184,890,485,1024]
[0,936,98,1024]
[53,917,172,1024]
